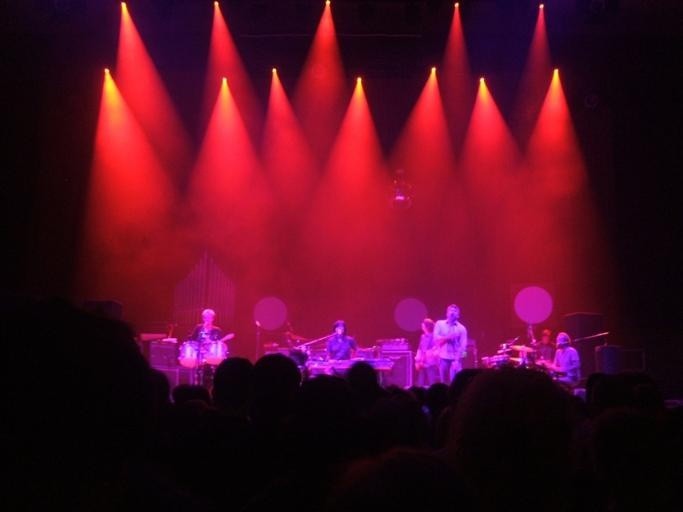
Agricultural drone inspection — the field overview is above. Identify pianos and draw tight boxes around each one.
[305,360,396,374]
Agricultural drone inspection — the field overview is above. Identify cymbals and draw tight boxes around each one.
[510,357,534,364]
[510,345,533,352]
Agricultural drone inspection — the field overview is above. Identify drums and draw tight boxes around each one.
[200,341,227,365]
[177,340,201,368]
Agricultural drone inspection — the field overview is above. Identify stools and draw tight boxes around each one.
[572,388,587,400]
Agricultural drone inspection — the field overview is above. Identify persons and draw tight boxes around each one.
[432,303,468,385]
[591,329,621,374]
[411,318,438,386]
[186,308,222,344]
[0,292,163,512]
[534,327,555,363]
[551,332,580,385]
[324,320,361,360]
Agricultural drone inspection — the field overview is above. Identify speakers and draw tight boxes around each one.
[379,351,413,391]
